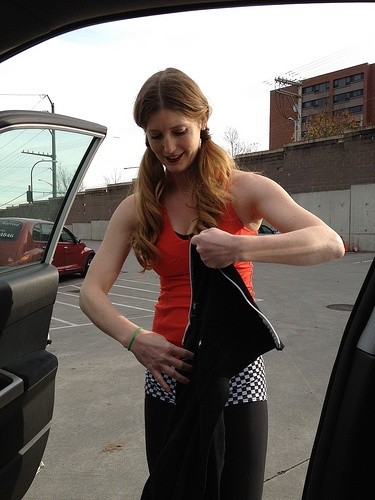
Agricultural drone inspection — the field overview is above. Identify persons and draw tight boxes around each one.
[81,68,345,500]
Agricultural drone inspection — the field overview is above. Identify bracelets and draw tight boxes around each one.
[126,326,142,350]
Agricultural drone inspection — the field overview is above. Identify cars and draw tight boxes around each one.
[0,217,97,277]
